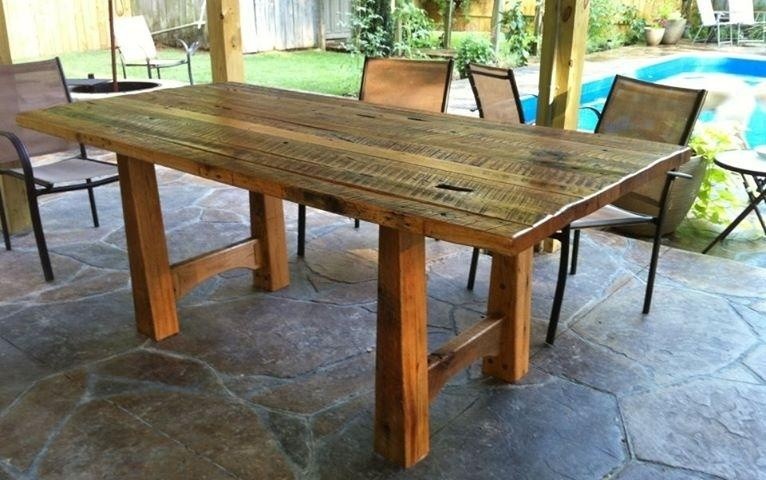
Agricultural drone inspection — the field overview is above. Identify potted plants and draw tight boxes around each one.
[610,123,741,238]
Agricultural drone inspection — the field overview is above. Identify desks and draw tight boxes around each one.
[702,150,766,255]
[13,79,691,470]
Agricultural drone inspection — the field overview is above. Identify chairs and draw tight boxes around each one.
[296,55,453,258]
[113,15,194,86]
[690,0,765,48]
[465,74,709,347]
[0,57,121,283]
[466,62,538,129]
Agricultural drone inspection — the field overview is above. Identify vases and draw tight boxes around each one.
[660,18,688,44]
[644,27,665,46]
